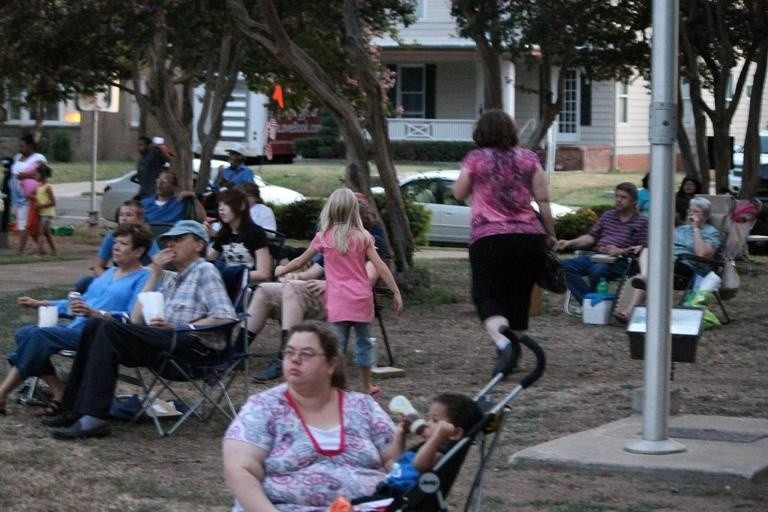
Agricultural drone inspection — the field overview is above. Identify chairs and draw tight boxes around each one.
[19,254,156,416]
[612,194,761,331]
[136,263,257,440]
[349,232,395,367]
[263,228,287,273]
[562,185,646,322]
[148,221,176,252]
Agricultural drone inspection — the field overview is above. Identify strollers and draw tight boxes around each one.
[331,325,547,511]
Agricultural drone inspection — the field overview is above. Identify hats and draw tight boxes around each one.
[224,142,248,157]
[158,220,210,246]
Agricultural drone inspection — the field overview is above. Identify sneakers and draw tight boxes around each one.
[493,344,513,378]
[249,357,283,384]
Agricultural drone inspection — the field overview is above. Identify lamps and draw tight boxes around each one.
[625,306,704,414]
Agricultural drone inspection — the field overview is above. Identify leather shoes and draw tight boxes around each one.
[36,399,112,441]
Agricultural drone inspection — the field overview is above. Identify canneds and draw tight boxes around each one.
[66,291,82,316]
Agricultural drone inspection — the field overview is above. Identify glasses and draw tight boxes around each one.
[282,348,328,361]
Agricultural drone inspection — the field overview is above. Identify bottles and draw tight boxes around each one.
[388,394,431,437]
[541,291,550,313]
[596,275,608,293]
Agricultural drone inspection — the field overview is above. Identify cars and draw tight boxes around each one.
[99,159,303,225]
[371,171,581,245]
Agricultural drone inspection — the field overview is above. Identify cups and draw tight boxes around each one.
[66,290,79,316]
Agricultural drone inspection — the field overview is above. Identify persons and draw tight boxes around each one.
[450,109,558,381]
[232,187,404,394]
[8,134,60,257]
[353,392,483,512]
[221,319,400,511]
[558,168,727,328]
[1,202,237,438]
[132,137,276,285]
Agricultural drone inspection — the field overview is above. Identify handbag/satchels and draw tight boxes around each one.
[536,251,567,294]
[722,261,740,297]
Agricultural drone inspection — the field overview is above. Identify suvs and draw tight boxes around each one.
[728,130,768,193]
